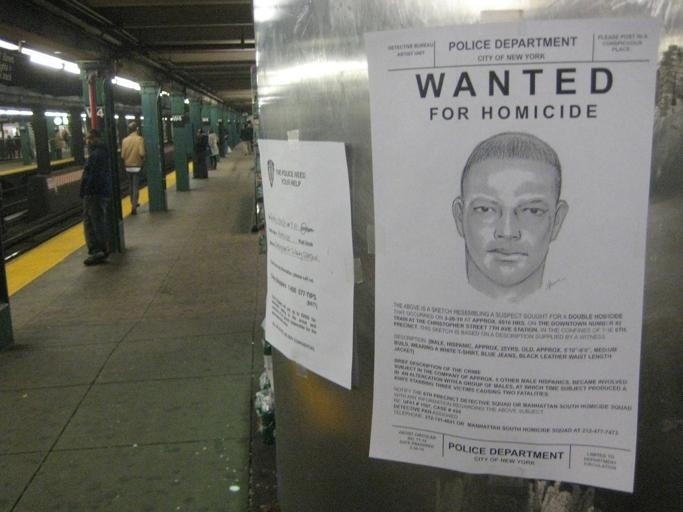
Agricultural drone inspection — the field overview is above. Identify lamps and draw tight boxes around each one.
[0,36,143,94]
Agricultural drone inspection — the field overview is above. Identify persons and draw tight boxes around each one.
[118,122,145,215]
[78,127,115,265]
[0,119,253,170]
[450,133,568,301]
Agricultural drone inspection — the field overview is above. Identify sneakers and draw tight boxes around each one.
[84,251,106,265]
